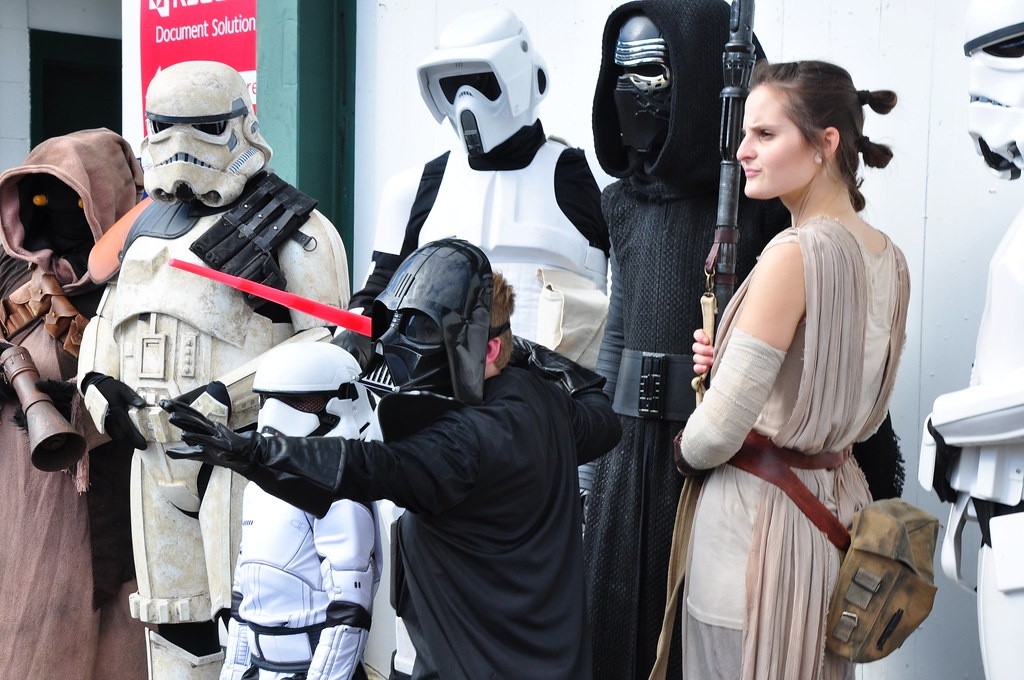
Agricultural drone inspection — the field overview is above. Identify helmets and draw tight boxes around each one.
[961,2,1023,182]
[254,344,368,453]
[140,61,274,207]
[420,7,547,154]
[613,19,671,148]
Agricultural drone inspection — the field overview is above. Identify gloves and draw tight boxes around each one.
[81,371,148,450]
[161,383,228,436]
[14,378,72,429]
[507,335,606,401]
[927,423,960,507]
[160,397,348,522]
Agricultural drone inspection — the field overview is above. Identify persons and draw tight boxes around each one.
[0,124,149,679]
[158,236,624,679]
[918,1,1023,679]
[75,60,355,680]
[217,341,377,679]
[677,61,912,680]
[575,0,904,679]
[331,8,611,680]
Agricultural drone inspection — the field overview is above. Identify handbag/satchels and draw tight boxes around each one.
[826,501,944,659]
